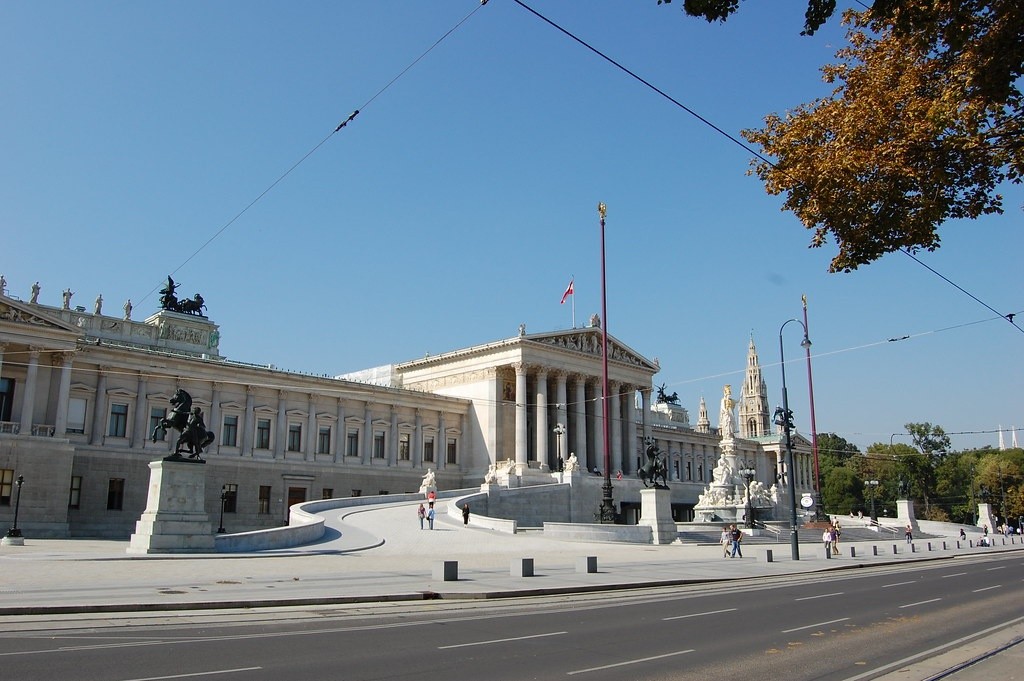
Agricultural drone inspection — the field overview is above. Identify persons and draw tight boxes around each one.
[557,456,564,472]
[652,455,668,486]
[960,529,966,540]
[462,503,469,527]
[1016,528,1021,535]
[593,466,602,477]
[484,465,498,484]
[905,525,913,544]
[417,503,427,530]
[976,524,990,547]
[832,516,841,539]
[427,505,435,530]
[428,491,436,509]
[857,510,864,520]
[850,511,853,519]
[822,527,840,559]
[421,468,436,486]
[996,522,1014,538]
[0,275,133,327]
[720,524,743,559]
[158,319,206,345]
[170,407,204,461]
[695,384,771,506]
[563,452,579,471]
[616,470,622,480]
[503,457,516,475]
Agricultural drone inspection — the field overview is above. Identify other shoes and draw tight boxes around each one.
[464,525,467,528]
[832,553,836,555]
[837,550,839,554]
[730,556,734,558]
[740,555,742,558]
[722,556,726,558]
[729,552,730,556]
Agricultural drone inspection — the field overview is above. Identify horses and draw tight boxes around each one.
[637,445,659,488]
[179,293,208,316]
[151,388,215,458]
[665,392,681,404]
[898,473,908,494]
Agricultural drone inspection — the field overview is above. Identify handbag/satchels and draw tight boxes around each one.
[426,517,429,520]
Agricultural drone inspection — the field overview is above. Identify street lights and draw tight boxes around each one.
[7,475,25,537]
[970,462,977,525]
[218,485,226,533]
[552,426,566,471]
[772,319,814,560]
[864,480,879,527]
[738,464,758,529]
[998,466,1008,526]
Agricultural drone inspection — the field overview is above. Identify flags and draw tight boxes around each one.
[560,274,575,304]
